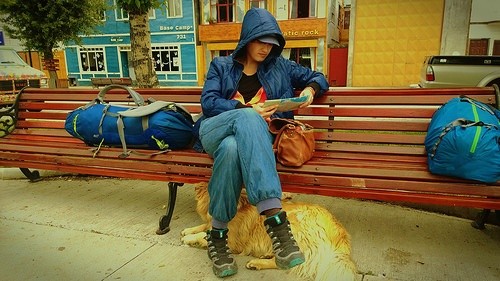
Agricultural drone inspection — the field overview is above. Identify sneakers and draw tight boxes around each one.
[203,228,239,278]
[263,211,305,269]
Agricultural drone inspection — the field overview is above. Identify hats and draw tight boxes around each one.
[259,35,281,47]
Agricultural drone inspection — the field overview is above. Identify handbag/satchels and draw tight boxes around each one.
[267,118,317,167]
[65,83,195,149]
[426,96,500,183]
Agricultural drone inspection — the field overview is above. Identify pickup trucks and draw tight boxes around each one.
[418,53,500,110]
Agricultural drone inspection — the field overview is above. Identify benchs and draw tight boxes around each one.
[91,77,133,88]
[0,83,500,235]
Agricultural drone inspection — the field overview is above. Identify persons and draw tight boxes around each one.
[194,8,329,276]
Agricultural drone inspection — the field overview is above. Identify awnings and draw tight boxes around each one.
[0,50,26,65]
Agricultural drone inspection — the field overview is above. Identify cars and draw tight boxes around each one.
[0,46,47,112]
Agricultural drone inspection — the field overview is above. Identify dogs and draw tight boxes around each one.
[181,181,360,280]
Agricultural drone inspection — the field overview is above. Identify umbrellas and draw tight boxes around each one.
[0,60,46,95]
